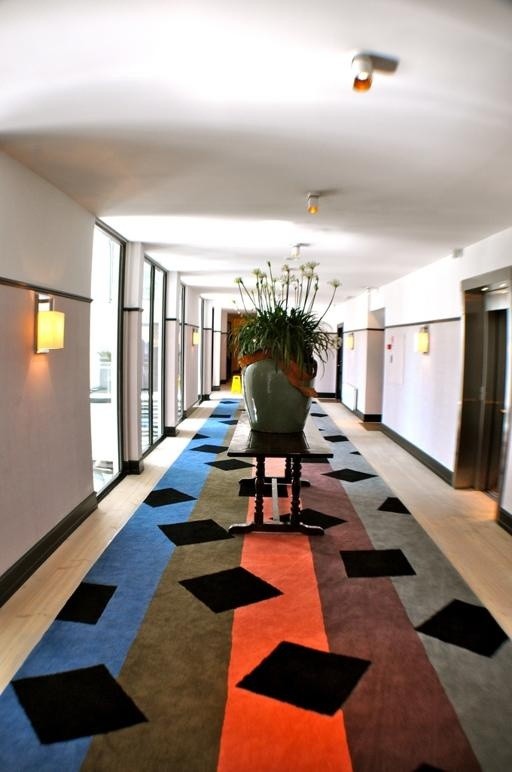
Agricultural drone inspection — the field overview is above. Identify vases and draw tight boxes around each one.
[243,355,311,435]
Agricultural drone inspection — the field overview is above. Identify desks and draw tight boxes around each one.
[227,411,334,537]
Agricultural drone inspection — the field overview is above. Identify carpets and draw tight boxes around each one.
[0,397,510,768]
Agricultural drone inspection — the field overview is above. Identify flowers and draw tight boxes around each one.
[227,261,341,382]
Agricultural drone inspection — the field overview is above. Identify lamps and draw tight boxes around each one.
[35,292,65,353]
[306,192,320,214]
[417,325,429,352]
